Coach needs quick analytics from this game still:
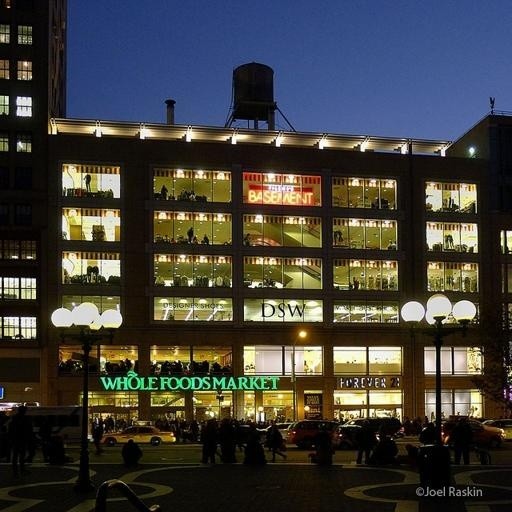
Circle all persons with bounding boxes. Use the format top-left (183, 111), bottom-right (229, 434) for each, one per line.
top-left (416, 424), bottom-right (469, 512)
top-left (85, 174), bottom-right (92, 193)
top-left (160, 185), bottom-right (169, 200)
top-left (188, 227), bottom-right (194, 244)
top-left (1, 402), bottom-right (472, 472)
top-left (106, 356), bottom-right (232, 379)
top-left (204, 234), bottom-right (210, 244)
top-left (243, 233), bottom-right (253, 247)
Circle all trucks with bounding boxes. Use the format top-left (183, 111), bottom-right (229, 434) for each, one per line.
top-left (0, 404), bottom-right (87, 449)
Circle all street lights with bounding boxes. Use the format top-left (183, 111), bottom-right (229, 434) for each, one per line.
top-left (292, 328), bottom-right (310, 421)
top-left (48, 301), bottom-right (125, 496)
top-left (401, 293), bottom-right (478, 444)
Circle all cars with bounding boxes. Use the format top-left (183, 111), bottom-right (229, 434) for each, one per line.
top-left (234, 416), bottom-right (409, 451)
top-left (417, 415), bottom-right (512, 447)
top-left (98, 420), bottom-right (177, 447)
